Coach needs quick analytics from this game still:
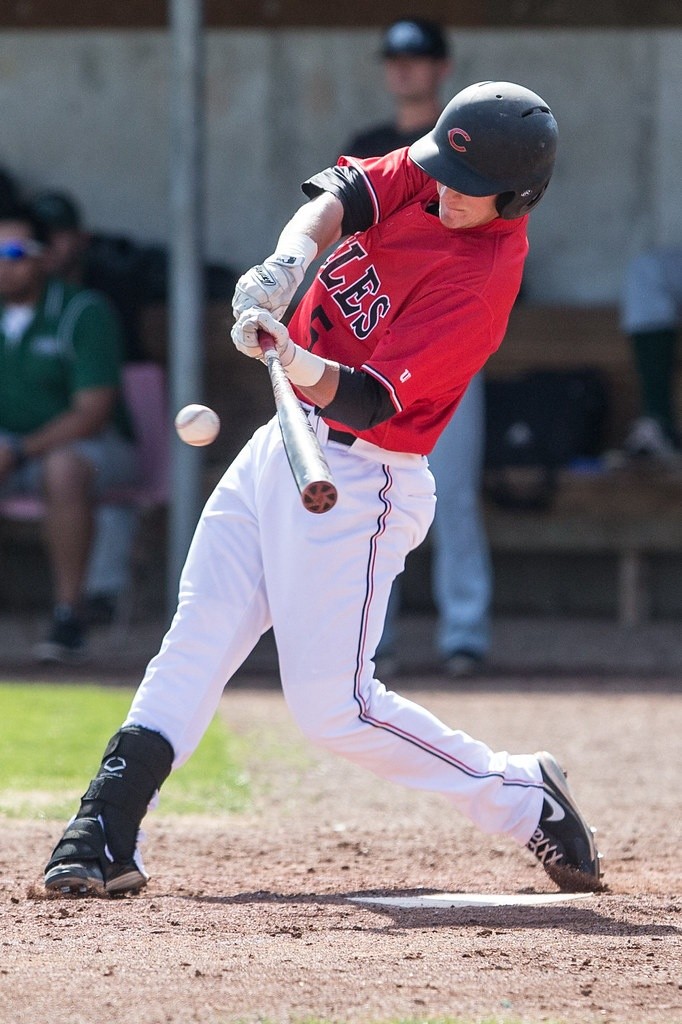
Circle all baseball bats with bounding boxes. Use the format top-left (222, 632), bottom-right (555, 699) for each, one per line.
top-left (256, 327), bottom-right (339, 516)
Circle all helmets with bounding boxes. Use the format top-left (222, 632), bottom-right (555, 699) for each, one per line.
top-left (406, 81), bottom-right (559, 220)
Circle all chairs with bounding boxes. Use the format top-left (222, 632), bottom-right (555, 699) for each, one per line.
top-left (0, 363), bottom-right (169, 639)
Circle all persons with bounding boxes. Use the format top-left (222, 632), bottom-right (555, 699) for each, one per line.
top-left (619, 233), bottom-right (682, 471)
top-left (0, 156), bottom-right (258, 674)
top-left (41, 78), bottom-right (604, 899)
top-left (333, 16), bottom-right (495, 678)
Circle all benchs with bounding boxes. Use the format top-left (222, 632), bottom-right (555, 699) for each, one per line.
top-left (138, 300), bottom-right (682, 627)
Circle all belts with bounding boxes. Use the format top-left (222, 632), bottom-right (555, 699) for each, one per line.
top-left (303, 409), bottom-right (356, 446)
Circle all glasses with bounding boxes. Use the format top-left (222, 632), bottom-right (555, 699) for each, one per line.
top-left (3, 241), bottom-right (43, 265)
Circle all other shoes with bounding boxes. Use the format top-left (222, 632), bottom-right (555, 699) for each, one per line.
top-left (31, 613), bottom-right (97, 667)
top-left (445, 649), bottom-right (483, 681)
top-left (374, 648), bottom-right (410, 681)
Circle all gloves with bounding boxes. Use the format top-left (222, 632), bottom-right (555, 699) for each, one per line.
top-left (230, 252), bottom-right (306, 318)
top-left (231, 307), bottom-right (298, 376)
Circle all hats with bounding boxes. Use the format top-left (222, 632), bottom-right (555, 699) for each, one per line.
top-left (376, 17), bottom-right (450, 62)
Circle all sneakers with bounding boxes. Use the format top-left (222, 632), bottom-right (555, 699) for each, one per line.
top-left (509, 750), bottom-right (604, 892)
top-left (44, 725), bottom-right (174, 894)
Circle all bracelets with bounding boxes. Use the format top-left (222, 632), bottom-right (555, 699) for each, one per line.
top-left (282, 343), bottom-right (325, 386)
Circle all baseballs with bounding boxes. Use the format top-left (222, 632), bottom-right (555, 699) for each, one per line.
top-left (173, 404), bottom-right (220, 448)
